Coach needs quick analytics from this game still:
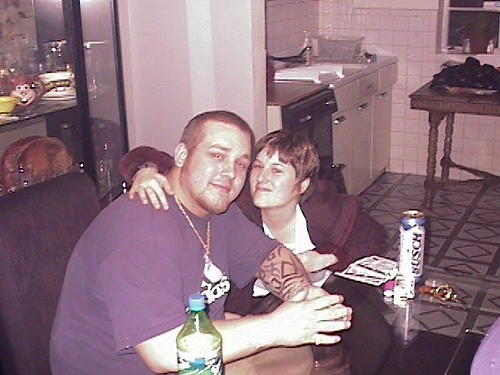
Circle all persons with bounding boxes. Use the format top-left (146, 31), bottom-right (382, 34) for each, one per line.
top-left (118, 130), bottom-right (394, 375)
top-left (49, 109), bottom-right (354, 375)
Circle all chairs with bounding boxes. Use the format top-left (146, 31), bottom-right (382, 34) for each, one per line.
top-left (1, 173), bottom-right (103, 375)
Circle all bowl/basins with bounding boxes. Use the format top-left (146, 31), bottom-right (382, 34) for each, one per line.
top-left (37, 72), bottom-right (70, 98)
top-left (0, 96), bottom-right (23, 113)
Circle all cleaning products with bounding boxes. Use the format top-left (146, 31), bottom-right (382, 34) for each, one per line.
top-left (301, 30), bottom-right (312, 66)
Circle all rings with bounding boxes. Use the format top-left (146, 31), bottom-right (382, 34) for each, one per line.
top-left (316, 333), bottom-right (321, 346)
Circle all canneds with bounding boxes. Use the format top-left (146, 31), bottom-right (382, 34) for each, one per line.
top-left (397, 209), bottom-right (426, 279)
top-left (395, 284), bottom-right (421, 345)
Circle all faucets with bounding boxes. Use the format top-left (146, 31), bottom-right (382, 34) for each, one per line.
top-left (286, 47), bottom-right (311, 67)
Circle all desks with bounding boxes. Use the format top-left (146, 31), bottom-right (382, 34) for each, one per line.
top-left (409, 82), bottom-right (500, 210)
top-left (171, 258), bottom-right (500, 374)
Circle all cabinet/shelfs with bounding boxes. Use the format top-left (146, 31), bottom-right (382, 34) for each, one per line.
top-left (0, 0), bottom-right (130, 208)
top-left (267, 63), bottom-right (398, 196)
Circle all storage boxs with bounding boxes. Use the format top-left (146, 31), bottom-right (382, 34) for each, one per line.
top-left (307, 34), bottom-right (364, 62)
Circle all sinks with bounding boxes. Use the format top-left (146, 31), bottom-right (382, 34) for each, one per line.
top-left (272, 61), bottom-right (367, 85)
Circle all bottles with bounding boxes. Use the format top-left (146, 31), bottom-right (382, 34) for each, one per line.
top-left (175, 293), bottom-right (224, 375)
top-left (463, 39), bottom-right (471, 54)
top-left (486, 40), bottom-right (494, 53)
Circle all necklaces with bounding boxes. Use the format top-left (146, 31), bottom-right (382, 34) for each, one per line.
top-left (173, 194), bottom-right (223, 283)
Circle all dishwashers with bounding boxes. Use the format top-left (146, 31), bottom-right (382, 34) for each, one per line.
top-left (281, 89), bottom-right (348, 198)
